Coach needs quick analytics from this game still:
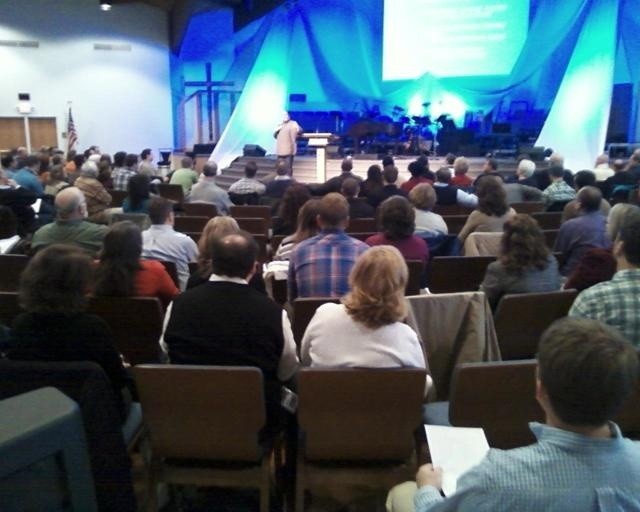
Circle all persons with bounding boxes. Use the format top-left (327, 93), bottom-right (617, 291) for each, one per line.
top-left (1, 111), bottom-right (640, 512)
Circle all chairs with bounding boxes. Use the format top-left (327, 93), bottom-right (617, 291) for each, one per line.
top-left (297, 367), bottom-right (419, 508)
top-left (425, 360), bottom-right (538, 449)
top-left (134, 367), bottom-right (271, 508)
top-left (0, 360), bottom-right (142, 511)
